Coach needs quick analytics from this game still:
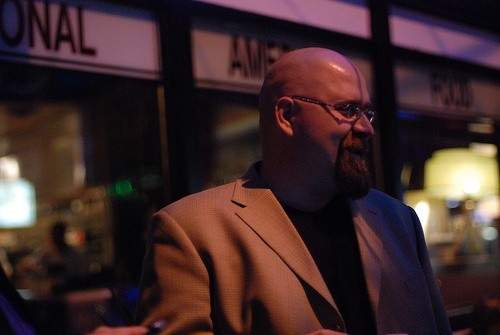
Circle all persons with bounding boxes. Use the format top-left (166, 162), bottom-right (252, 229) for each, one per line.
top-left (138, 46), bottom-right (457, 335)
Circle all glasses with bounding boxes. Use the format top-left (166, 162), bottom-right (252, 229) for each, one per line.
top-left (276, 95), bottom-right (378, 124)
